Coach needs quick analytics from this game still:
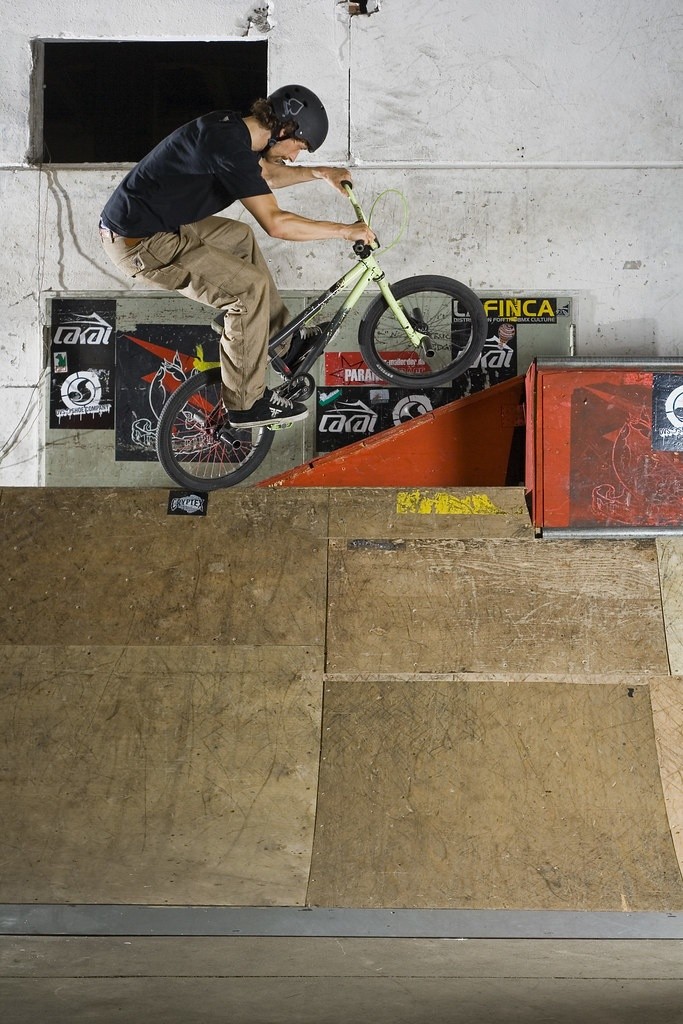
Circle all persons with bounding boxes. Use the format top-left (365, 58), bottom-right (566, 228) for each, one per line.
top-left (95, 86), bottom-right (379, 429)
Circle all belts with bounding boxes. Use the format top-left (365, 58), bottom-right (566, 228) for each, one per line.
top-left (99, 228), bottom-right (122, 238)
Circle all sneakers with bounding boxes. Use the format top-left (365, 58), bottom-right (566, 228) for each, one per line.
top-left (270, 321), bottom-right (340, 375)
top-left (228, 386), bottom-right (309, 428)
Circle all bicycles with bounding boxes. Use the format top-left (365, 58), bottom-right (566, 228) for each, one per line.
top-left (156, 166), bottom-right (489, 493)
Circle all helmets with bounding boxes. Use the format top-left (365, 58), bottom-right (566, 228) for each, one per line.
top-left (268, 85), bottom-right (329, 153)
